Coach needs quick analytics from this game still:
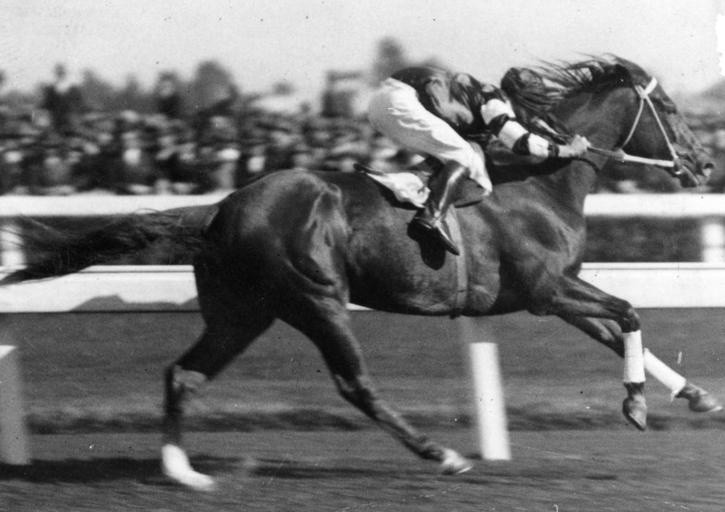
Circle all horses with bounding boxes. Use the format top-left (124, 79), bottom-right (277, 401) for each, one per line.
top-left (0, 50), bottom-right (725, 492)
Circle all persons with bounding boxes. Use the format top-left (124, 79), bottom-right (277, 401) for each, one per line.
top-left (587, 113), bottom-right (725, 193)
top-left (367, 66), bottom-right (592, 255)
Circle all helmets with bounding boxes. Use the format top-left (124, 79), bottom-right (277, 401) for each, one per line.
top-left (500, 65), bottom-right (546, 113)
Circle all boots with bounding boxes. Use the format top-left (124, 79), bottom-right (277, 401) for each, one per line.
top-left (408, 159), bottom-right (472, 257)
